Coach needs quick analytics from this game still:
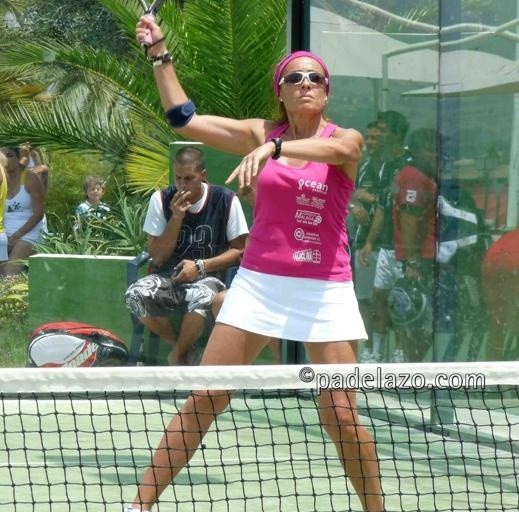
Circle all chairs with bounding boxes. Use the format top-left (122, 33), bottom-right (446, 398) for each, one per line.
top-left (126, 250), bottom-right (241, 366)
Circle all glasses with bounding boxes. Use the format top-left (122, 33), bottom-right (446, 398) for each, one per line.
top-left (278, 70), bottom-right (326, 87)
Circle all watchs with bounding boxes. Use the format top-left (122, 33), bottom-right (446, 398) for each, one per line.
top-left (271, 137), bottom-right (283, 159)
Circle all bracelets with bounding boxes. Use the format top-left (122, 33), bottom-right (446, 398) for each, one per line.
top-left (149, 49), bottom-right (172, 68)
top-left (194, 259), bottom-right (209, 278)
top-left (146, 37), bottom-right (165, 50)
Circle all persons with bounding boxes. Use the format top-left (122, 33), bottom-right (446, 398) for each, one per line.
top-left (0, 147), bottom-right (49, 282)
top-left (73, 175), bottom-right (112, 230)
top-left (213, 289), bottom-right (282, 363)
top-left (122, 10), bottom-right (390, 511)
top-left (18, 140), bottom-right (50, 175)
top-left (347, 107), bottom-right (518, 426)
top-left (0, 151), bottom-right (10, 270)
top-left (123, 145), bottom-right (252, 373)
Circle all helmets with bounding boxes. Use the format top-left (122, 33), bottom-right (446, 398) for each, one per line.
top-left (386, 278), bottom-right (430, 333)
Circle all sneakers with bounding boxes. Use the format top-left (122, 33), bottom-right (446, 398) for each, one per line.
top-left (359, 349), bottom-right (405, 363)
